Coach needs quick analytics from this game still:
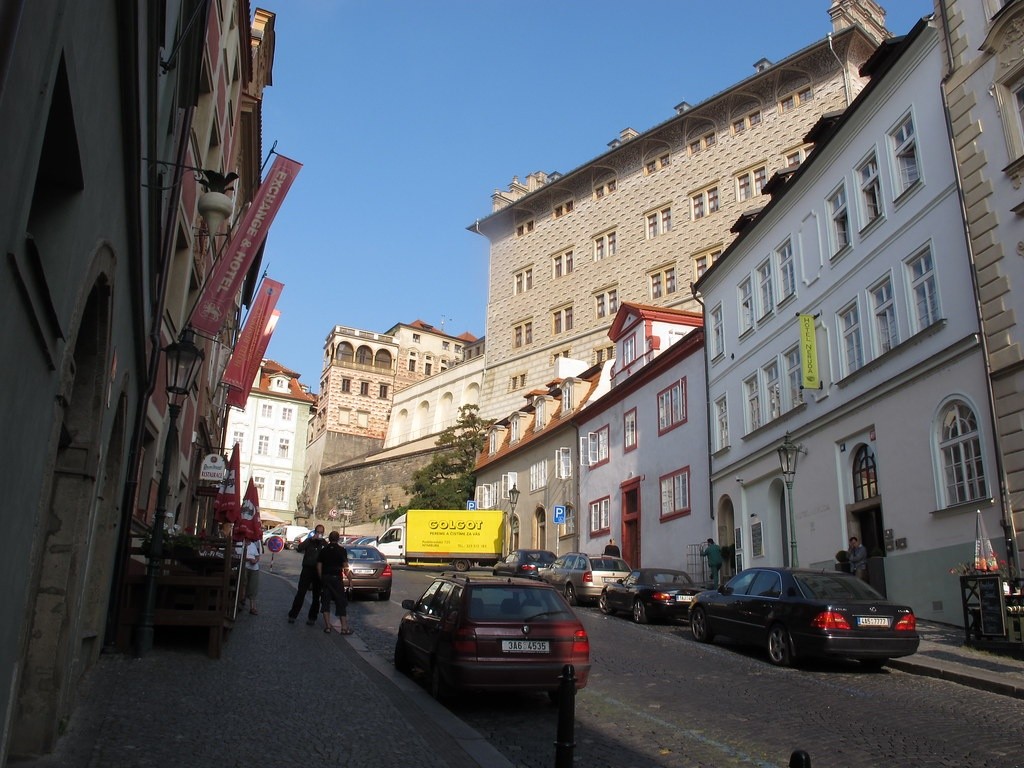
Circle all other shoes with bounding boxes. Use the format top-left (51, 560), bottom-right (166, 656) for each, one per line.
top-left (288, 616), bottom-right (296, 623)
top-left (250, 608), bottom-right (259, 615)
top-left (307, 620), bottom-right (316, 625)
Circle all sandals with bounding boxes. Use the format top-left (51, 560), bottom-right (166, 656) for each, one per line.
top-left (325, 627), bottom-right (331, 633)
top-left (341, 628), bottom-right (354, 635)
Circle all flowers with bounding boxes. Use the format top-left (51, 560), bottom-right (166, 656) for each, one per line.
top-left (947, 552), bottom-right (1015, 577)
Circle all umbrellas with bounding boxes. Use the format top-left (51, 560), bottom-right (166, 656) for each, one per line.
top-left (215, 442), bottom-right (263, 619)
top-left (973, 509), bottom-right (998, 574)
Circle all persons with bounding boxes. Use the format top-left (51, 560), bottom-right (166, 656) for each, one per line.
top-left (316, 531), bottom-right (355, 635)
top-left (604, 539), bottom-right (620, 559)
top-left (288, 525), bottom-right (328, 625)
top-left (699, 538), bottom-right (722, 591)
top-left (238, 541), bottom-right (260, 616)
top-left (846, 536), bottom-right (868, 581)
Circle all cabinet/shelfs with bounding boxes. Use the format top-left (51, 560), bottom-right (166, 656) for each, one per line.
top-left (119, 534), bottom-right (230, 660)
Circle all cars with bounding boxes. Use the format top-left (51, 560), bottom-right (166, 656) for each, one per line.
top-left (339, 543), bottom-right (392, 600)
top-left (537, 552), bottom-right (633, 607)
top-left (598, 568), bottom-right (707, 624)
top-left (492, 549), bottom-right (558, 582)
top-left (391, 572), bottom-right (591, 709)
top-left (689, 566), bottom-right (921, 676)
top-left (262, 524), bottom-right (375, 550)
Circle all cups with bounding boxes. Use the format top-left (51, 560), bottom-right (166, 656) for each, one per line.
top-left (316, 534), bottom-right (322, 540)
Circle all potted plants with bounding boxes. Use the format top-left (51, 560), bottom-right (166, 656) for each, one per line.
top-left (198, 169), bottom-right (238, 242)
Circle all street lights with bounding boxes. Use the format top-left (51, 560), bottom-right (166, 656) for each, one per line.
top-left (777, 431), bottom-right (800, 566)
top-left (382, 495), bottom-right (391, 531)
top-left (129, 320), bottom-right (206, 660)
top-left (507, 483), bottom-right (521, 551)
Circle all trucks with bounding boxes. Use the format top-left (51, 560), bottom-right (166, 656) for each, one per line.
top-left (367, 509), bottom-right (507, 572)
